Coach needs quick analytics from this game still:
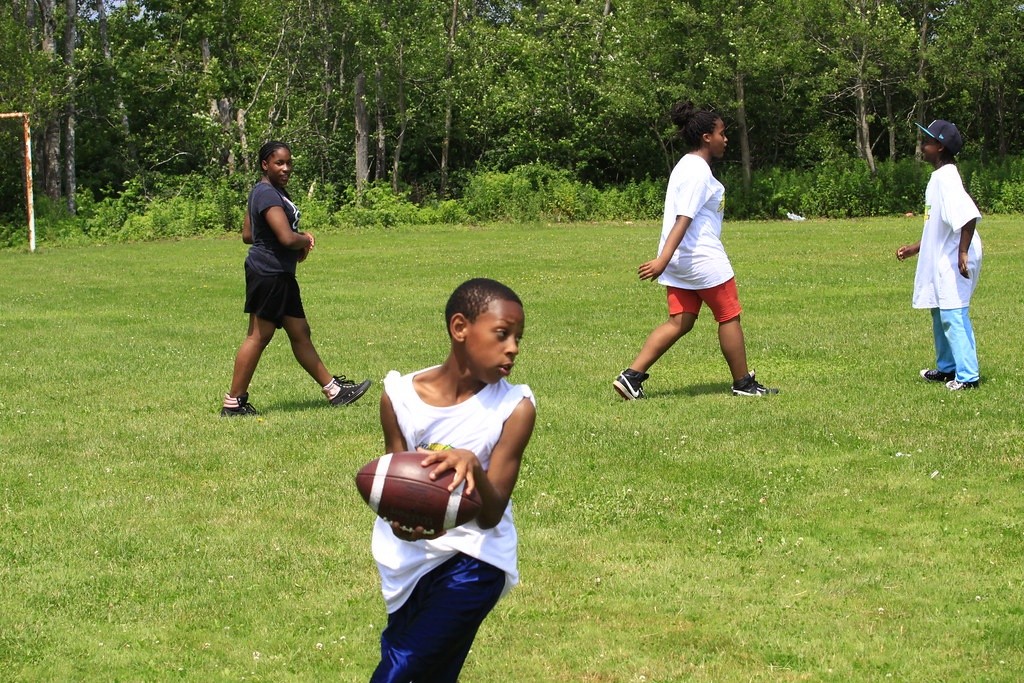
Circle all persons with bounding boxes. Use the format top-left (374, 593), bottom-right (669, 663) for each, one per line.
top-left (896, 121), bottom-right (983, 392)
top-left (611, 100), bottom-right (778, 400)
top-left (367, 278), bottom-right (537, 682)
top-left (219, 141), bottom-right (370, 416)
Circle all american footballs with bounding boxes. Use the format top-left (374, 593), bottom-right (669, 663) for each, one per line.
top-left (354, 452), bottom-right (482, 535)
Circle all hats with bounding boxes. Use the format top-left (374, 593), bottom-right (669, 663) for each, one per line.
top-left (915, 119), bottom-right (966, 156)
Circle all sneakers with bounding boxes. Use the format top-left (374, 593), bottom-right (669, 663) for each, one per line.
top-left (612, 369), bottom-right (648, 401)
top-left (323, 376), bottom-right (373, 407)
top-left (920, 367), bottom-right (956, 382)
top-left (943, 380), bottom-right (980, 389)
top-left (219, 393), bottom-right (260, 417)
top-left (734, 369), bottom-right (779, 397)
